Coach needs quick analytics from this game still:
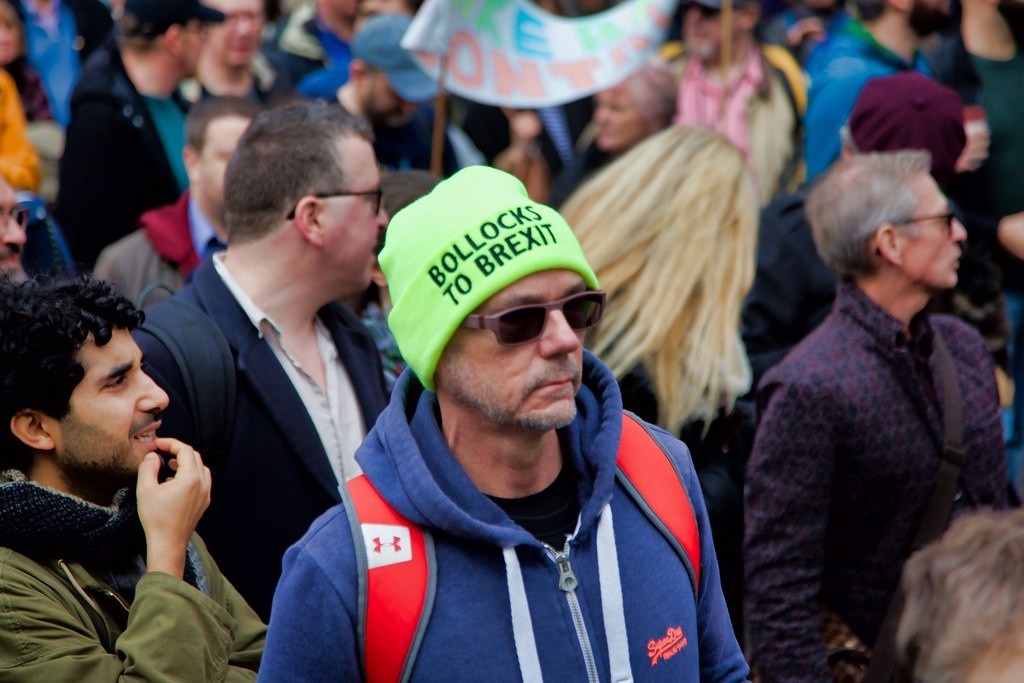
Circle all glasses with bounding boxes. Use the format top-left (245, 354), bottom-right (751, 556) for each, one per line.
top-left (683, 0), bottom-right (720, 16)
top-left (287, 185), bottom-right (384, 220)
top-left (11, 208), bottom-right (30, 231)
top-left (874, 198), bottom-right (959, 256)
top-left (461, 287), bottom-right (607, 347)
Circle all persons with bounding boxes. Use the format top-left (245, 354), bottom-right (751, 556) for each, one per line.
top-left (557, 124), bottom-right (754, 652)
top-left (0, 275), bottom-right (269, 683)
top-left (254, 164), bottom-right (750, 683)
top-left (131, 101), bottom-right (391, 626)
top-left (0, 0), bottom-right (1024, 410)
top-left (743, 150), bottom-right (1022, 683)
top-left (894, 504), bottom-right (1024, 683)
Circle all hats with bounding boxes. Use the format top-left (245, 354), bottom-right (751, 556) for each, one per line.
top-left (350, 13), bottom-right (448, 103)
top-left (123, 0), bottom-right (225, 39)
top-left (849, 71), bottom-right (967, 185)
top-left (390, 169), bottom-right (601, 394)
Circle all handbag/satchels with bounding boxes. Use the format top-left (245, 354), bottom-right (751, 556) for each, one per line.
top-left (740, 599), bottom-right (873, 683)
